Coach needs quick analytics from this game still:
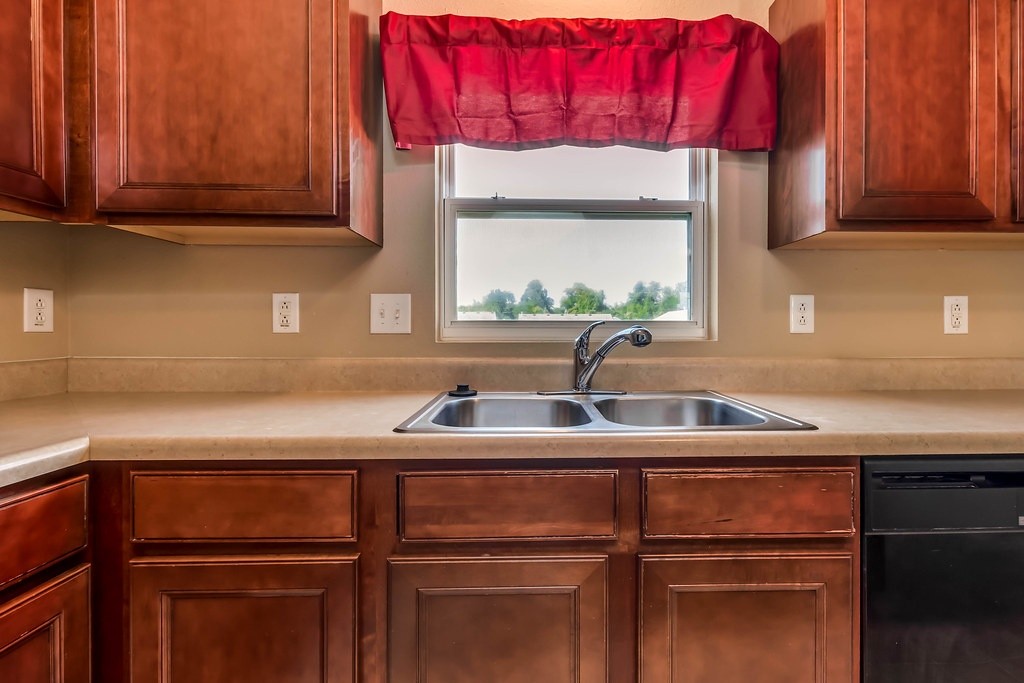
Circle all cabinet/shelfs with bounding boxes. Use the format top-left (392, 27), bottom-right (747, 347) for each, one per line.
top-left (767, 0), bottom-right (1024, 252)
top-left (118, 467), bottom-right (856, 683)
top-left (0, 0), bottom-right (384, 248)
top-left (0, 473), bottom-right (93, 683)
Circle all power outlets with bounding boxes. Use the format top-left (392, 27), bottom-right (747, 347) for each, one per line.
top-left (943, 296), bottom-right (968, 335)
top-left (272, 292), bottom-right (299, 334)
top-left (789, 295), bottom-right (814, 334)
top-left (371, 293), bottom-right (412, 334)
top-left (23, 287), bottom-right (54, 333)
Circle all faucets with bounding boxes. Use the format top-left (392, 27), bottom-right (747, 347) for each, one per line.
top-left (573, 320), bottom-right (652, 391)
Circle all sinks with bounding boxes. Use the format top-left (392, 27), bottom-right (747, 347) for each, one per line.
top-left (432, 396), bottom-right (591, 426)
top-left (595, 396), bottom-right (768, 428)
